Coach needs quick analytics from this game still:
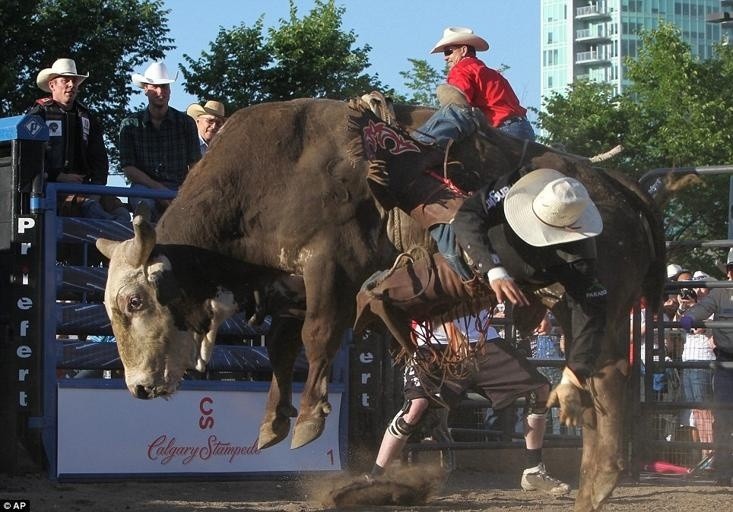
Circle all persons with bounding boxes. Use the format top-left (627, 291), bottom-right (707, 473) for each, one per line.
top-left (451, 149), bottom-right (666, 423)
top-left (530, 258), bottom-right (733, 488)
top-left (20, 59), bottom-right (133, 223)
top-left (115, 62), bottom-right (199, 222)
top-left (185, 101), bottom-right (227, 156)
top-left (358, 302), bottom-right (570, 495)
top-left (431, 28), bottom-right (532, 170)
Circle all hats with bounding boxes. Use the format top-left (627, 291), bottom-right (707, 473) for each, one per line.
top-left (186, 100), bottom-right (229, 124)
top-left (36, 58), bottom-right (89, 94)
top-left (502, 168), bottom-right (604, 248)
top-left (429, 26), bottom-right (490, 55)
top-left (130, 61), bottom-right (179, 89)
top-left (667, 263), bottom-right (717, 282)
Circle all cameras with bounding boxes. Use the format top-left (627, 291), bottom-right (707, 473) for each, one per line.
top-left (678, 290), bottom-right (698, 304)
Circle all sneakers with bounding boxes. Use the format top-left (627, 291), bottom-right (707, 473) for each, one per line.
top-left (434, 83), bottom-right (480, 129)
top-left (519, 463), bottom-right (572, 496)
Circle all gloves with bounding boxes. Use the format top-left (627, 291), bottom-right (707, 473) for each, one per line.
top-left (544, 366), bottom-right (590, 429)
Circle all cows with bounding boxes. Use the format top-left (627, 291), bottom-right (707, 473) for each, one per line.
top-left (95, 96), bottom-right (665, 512)
